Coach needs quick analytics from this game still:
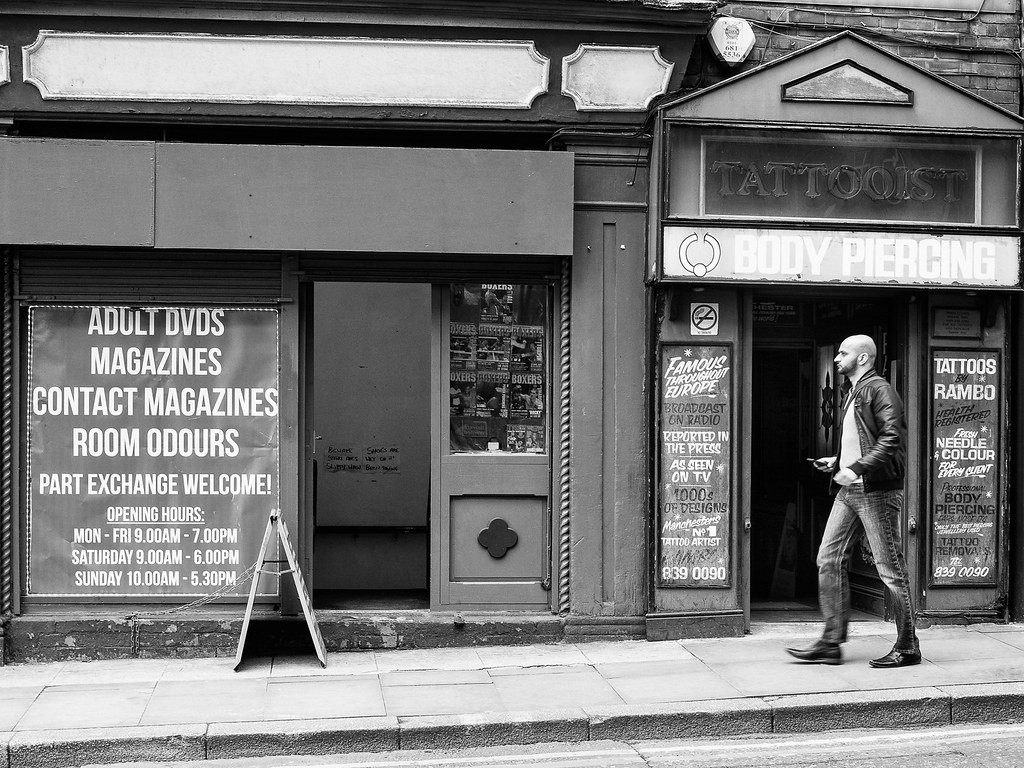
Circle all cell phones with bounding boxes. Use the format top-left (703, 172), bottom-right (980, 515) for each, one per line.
top-left (806, 458), bottom-right (834, 468)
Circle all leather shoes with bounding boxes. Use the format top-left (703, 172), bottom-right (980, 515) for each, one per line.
top-left (784, 641), bottom-right (843, 663)
top-left (868, 649), bottom-right (922, 668)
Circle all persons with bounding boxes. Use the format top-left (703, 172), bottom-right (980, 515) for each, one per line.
top-left (785, 335), bottom-right (922, 668)
